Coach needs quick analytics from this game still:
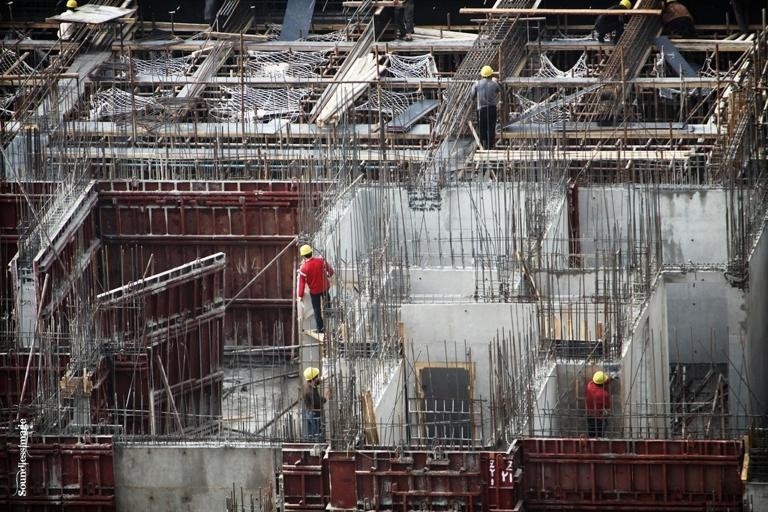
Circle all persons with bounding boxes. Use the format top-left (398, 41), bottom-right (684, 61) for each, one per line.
top-left (663, 0), bottom-right (698, 37)
top-left (471, 66), bottom-right (501, 150)
top-left (393, 0), bottom-right (415, 41)
top-left (57, 0), bottom-right (78, 42)
top-left (298, 245), bottom-right (333, 333)
top-left (587, 371), bottom-right (612, 437)
top-left (595, 0), bottom-right (632, 44)
top-left (303, 367), bottom-right (326, 443)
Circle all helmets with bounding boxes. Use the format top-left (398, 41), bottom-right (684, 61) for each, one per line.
top-left (620, 0), bottom-right (632, 9)
top-left (304, 367), bottom-right (320, 381)
top-left (480, 65), bottom-right (493, 77)
top-left (300, 245), bottom-right (313, 257)
top-left (67, 0), bottom-right (77, 8)
top-left (593, 370), bottom-right (607, 384)
